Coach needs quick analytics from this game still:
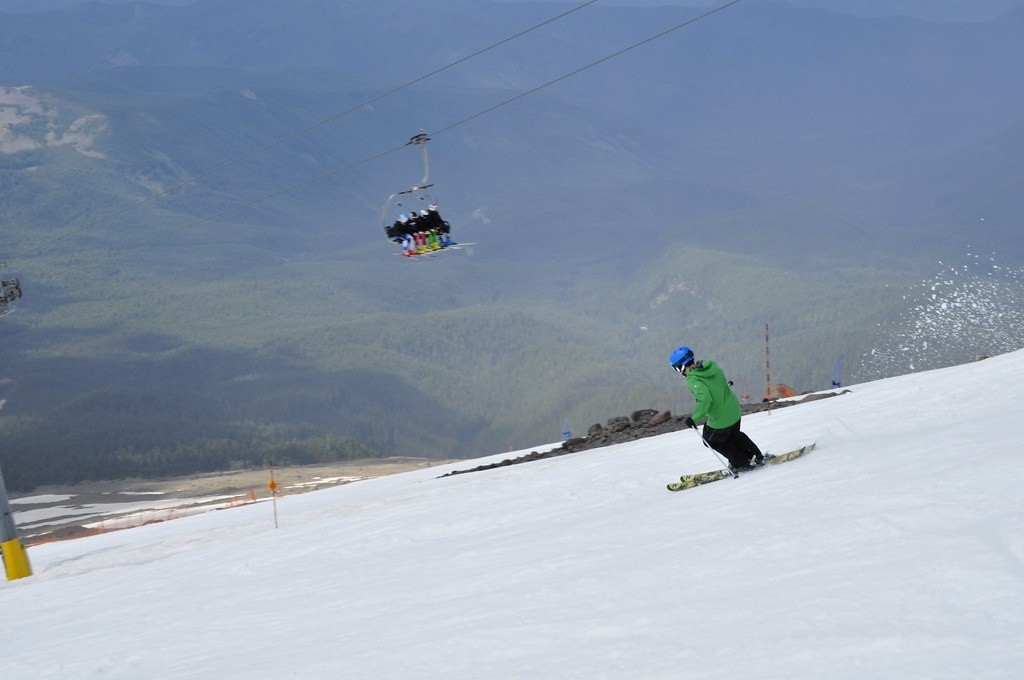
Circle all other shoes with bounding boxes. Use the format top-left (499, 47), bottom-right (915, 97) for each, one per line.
top-left (753, 454), bottom-right (764, 465)
top-left (403, 238), bottom-right (457, 256)
top-left (724, 461), bottom-right (749, 475)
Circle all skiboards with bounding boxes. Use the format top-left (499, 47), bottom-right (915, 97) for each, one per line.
top-left (393, 242), bottom-right (478, 261)
top-left (667, 442), bottom-right (816, 492)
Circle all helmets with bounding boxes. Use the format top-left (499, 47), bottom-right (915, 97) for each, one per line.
top-left (400, 203), bottom-right (438, 222)
top-left (668, 347), bottom-right (694, 368)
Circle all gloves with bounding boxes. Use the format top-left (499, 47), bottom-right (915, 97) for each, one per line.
top-left (685, 417), bottom-right (695, 427)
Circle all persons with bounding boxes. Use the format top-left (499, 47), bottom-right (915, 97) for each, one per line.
top-left (668, 346), bottom-right (764, 472)
top-left (417, 209), bottom-right (443, 250)
top-left (392, 214), bottom-right (417, 257)
top-left (407, 211), bottom-right (432, 254)
top-left (428, 203), bottom-right (457, 247)
